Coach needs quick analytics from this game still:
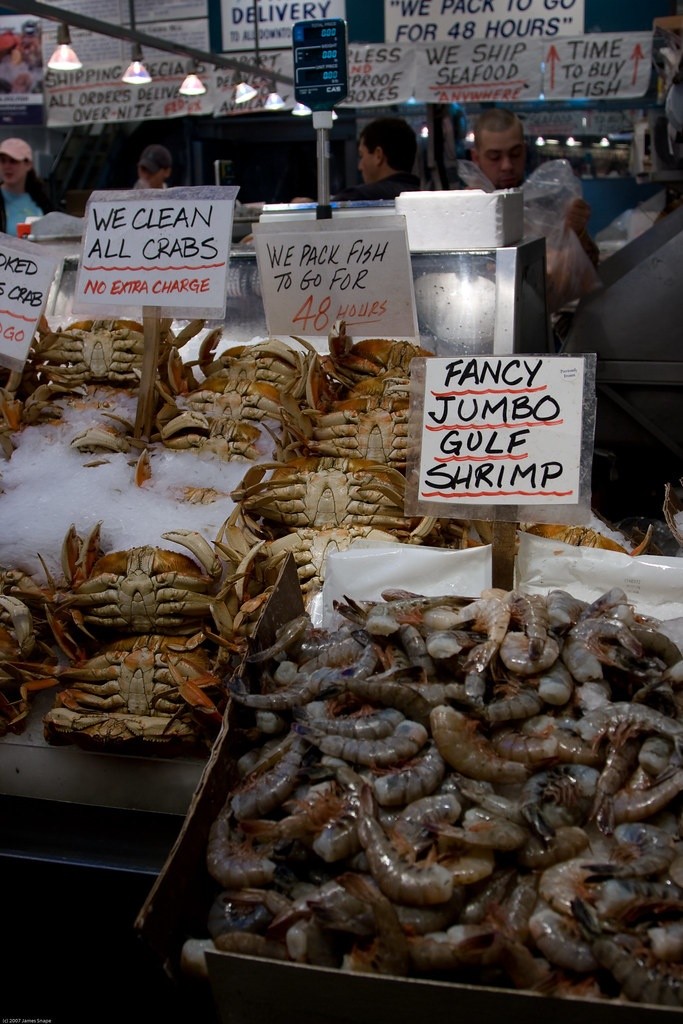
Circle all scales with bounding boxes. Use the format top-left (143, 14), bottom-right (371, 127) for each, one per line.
top-left (250, 19), bottom-right (396, 221)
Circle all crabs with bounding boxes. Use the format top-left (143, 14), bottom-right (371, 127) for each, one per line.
top-left (0, 315), bottom-right (206, 467)
top-left (520, 523), bottom-right (657, 556)
top-left (1, 520), bottom-right (233, 757)
top-left (208, 571), bottom-right (278, 653)
top-left (165, 323), bottom-right (494, 599)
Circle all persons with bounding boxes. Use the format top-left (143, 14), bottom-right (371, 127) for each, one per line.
top-left (131, 144), bottom-right (172, 190)
top-left (289, 115), bottom-right (422, 204)
top-left (0, 137), bottom-right (57, 241)
top-left (653, 52), bottom-right (683, 226)
top-left (456, 106), bottom-right (606, 355)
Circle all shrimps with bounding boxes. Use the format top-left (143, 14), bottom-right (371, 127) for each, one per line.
top-left (206, 589), bottom-right (683, 1009)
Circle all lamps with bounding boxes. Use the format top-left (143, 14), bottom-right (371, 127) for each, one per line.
top-left (179, 64), bottom-right (207, 95)
top-left (121, 46), bottom-right (153, 85)
top-left (48, 27), bottom-right (83, 71)
top-left (263, 87), bottom-right (285, 110)
top-left (291, 102), bottom-right (313, 116)
top-left (234, 76), bottom-right (257, 104)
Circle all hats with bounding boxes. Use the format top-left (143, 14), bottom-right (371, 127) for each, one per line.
top-left (138, 145), bottom-right (171, 173)
top-left (0, 137), bottom-right (32, 162)
